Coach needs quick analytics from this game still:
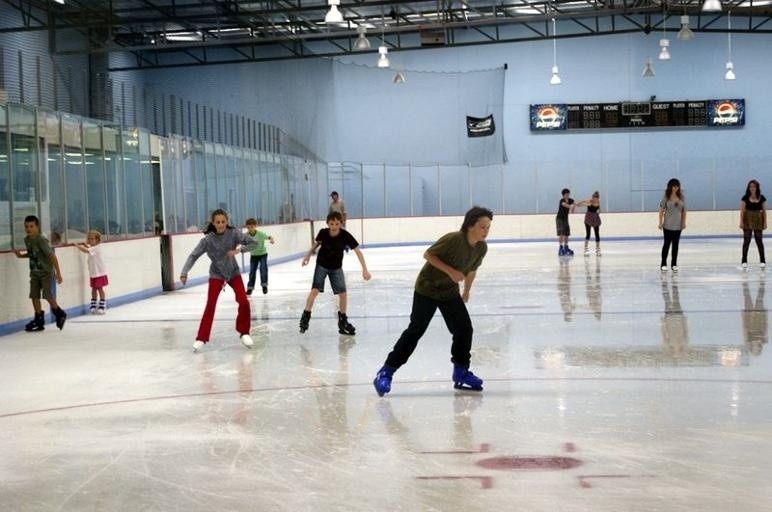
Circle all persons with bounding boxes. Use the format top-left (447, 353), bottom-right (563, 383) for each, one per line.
top-left (298, 210), bottom-right (374, 336)
top-left (554, 187), bottom-right (579, 257)
top-left (370, 203), bottom-right (495, 400)
top-left (280, 192), bottom-right (297, 223)
top-left (242, 216), bottom-right (276, 297)
top-left (656, 176), bottom-right (688, 272)
top-left (575, 190), bottom-right (604, 257)
top-left (326, 189), bottom-right (350, 254)
top-left (178, 207), bottom-right (260, 353)
top-left (72, 228), bottom-right (111, 315)
top-left (737, 178), bottom-right (769, 270)
top-left (69, 198), bottom-right (89, 227)
top-left (12, 214), bottom-right (69, 335)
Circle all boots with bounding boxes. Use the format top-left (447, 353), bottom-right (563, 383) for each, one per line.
top-left (25, 311), bottom-right (45, 331)
top-left (51, 305), bottom-right (66, 329)
top-left (337, 311), bottom-right (355, 334)
top-left (564, 245), bottom-right (573, 255)
top-left (300, 310), bottom-right (311, 330)
top-left (559, 245), bottom-right (565, 256)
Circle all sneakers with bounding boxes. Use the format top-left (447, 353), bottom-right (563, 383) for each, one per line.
top-left (98, 309), bottom-right (105, 314)
top-left (662, 266), bottom-right (668, 271)
top-left (90, 307), bottom-right (96, 313)
top-left (761, 263), bottom-right (765, 268)
top-left (193, 340), bottom-right (204, 350)
top-left (241, 334), bottom-right (252, 346)
top-left (376, 366), bottom-right (394, 394)
top-left (673, 266), bottom-right (679, 272)
top-left (263, 285), bottom-right (267, 294)
top-left (246, 289), bottom-right (252, 295)
top-left (743, 263), bottom-right (747, 268)
top-left (452, 367), bottom-right (483, 387)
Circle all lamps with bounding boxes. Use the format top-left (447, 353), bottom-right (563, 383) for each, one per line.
top-left (392, 68), bottom-right (408, 85)
top-left (724, 10), bottom-right (737, 80)
top-left (657, 0), bottom-right (672, 60)
top-left (377, 1), bottom-right (390, 69)
top-left (640, 1), bottom-right (655, 78)
top-left (353, 24), bottom-right (372, 49)
top-left (676, 1), bottom-right (695, 42)
top-left (549, 19), bottom-right (562, 85)
top-left (700, 1), bottom-right (723, 12)
top-left (324, 0), bottom-right (344, 22)
